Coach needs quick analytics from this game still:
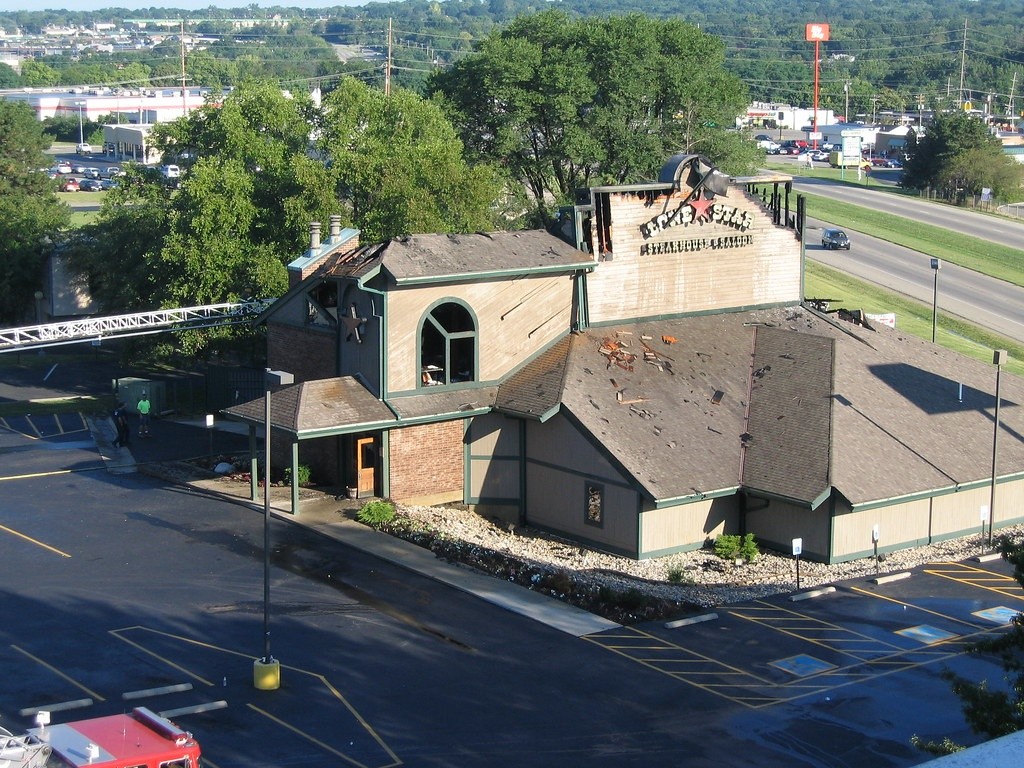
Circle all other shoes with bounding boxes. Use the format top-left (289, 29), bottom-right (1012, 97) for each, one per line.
top-left (120, 444), bottom-right (126, 447)
top-left (112, 441), bottom-right (117, 448)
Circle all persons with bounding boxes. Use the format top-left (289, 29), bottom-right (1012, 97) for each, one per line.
top-left (112, 402), bottom-right (129, 449)
top-left (137, 393), bottom-right (150, 434)
top-left (806, 153), bottom-right (812, 167)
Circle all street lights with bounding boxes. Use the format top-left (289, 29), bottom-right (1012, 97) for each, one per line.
top-left (931, 259), bottom-right (941, 343)
top-left (74, 101), bottom-right (86, 151)
top-left (261, 368), bottom-right (294, 663)
top-left (988, 349), bottom-right (1008, 544)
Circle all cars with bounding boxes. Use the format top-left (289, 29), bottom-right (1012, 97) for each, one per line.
top-left (167, 180), bottom-right (181, 189)
top-left (28, 160), bottom-right (145, 192)
top-left (754, 134), bottom-right (904, 168)
top-left (102, 141), bottom-right (115, 153)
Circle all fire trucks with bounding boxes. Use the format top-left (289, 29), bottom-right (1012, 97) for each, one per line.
top-left (0, 705), bottom-right (203, 768)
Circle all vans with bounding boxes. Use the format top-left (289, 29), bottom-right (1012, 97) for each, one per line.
top-left (160, 165), bottom-right (180, 180)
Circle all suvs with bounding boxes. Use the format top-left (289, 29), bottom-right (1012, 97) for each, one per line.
top-left (822, 228), bottom-right (850, 250)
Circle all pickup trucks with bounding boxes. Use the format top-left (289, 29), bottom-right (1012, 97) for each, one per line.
top-left (76, 143), bottom-right (92, 154)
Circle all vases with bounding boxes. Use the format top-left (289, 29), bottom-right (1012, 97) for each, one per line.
top-left (346, 485), bottom-right (358, 499)
